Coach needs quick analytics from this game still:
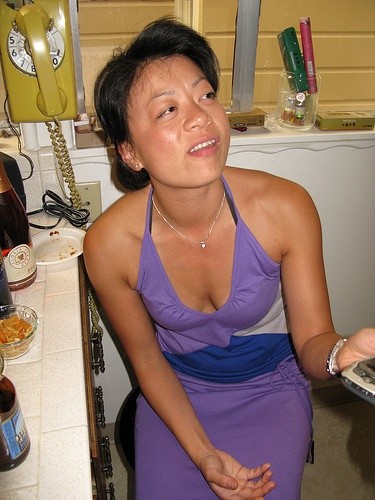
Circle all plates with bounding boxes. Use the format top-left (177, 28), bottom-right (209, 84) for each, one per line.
top-left (27, 228), bottom-right (87, 265)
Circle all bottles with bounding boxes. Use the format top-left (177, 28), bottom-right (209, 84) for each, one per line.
top-left (0, 151), bottom-right (37, 316)
top-left (0, 373), bottom-right (29, 472)
top-left (0, 248), bottom-right (15, 319)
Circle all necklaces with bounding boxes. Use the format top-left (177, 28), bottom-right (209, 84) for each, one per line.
top-left (151, 192), bottom-right (226, 248)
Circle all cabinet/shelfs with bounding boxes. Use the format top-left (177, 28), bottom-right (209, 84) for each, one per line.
top-left (0, 149), bottom-right (115, 500)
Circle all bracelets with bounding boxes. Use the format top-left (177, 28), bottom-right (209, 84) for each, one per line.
top-left (326, 338), bottom-right (348, 377)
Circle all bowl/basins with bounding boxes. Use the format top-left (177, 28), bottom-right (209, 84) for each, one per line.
top-left (0, 305), bottom-right (38, 359)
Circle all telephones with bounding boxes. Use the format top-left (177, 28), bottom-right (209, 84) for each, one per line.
top-left (0, 0), bottom-right (77, 124)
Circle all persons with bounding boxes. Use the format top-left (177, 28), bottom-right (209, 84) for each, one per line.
top-left (84, 16), bottom-right (375, 500)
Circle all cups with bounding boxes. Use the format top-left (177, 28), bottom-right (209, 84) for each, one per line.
top-left (276, 73), bottom-right (320, 132)
